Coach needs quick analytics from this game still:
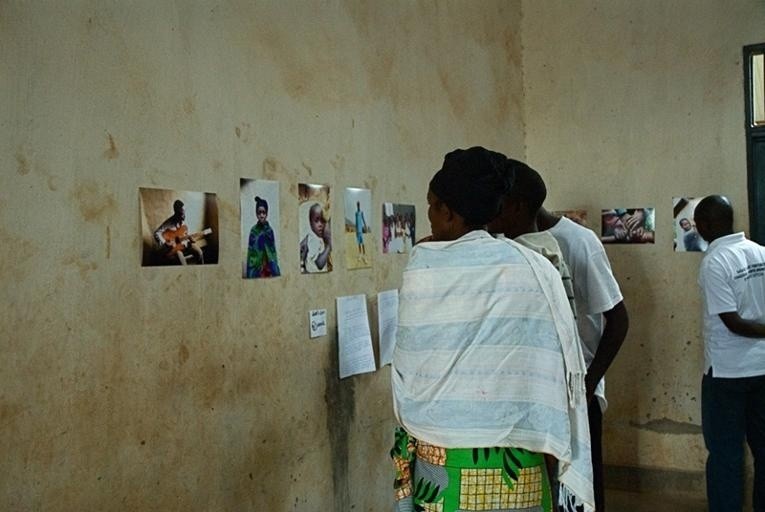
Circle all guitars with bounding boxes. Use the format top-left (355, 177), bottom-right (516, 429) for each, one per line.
top-left (162, 224), bottom-right (213, 257)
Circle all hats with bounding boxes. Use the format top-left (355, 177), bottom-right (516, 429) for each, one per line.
top-left (256, 200), bottom-right (268, 211)
top-left (429, 146), bottom-right (515, 225)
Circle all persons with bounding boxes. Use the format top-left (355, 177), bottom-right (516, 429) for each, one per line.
top-left (693, 194), bottom-right (765, 512)
top-left (536, 193), bottom-right (631, 512)
top-left (387, 147), bottom-right (583, 511)
top-left (300, 203), bottom-right (330, 271)
top-left (354, 201), bottom-right (369, 265)
top-left (602, 208), bottom-right (655, 243)
top-left (248, 196), bottom-right (278, 277)
top-left (489, 152), bottom-right (581, 321)
top-left (154, 199), bottom-right (203, 266)
top-left (383, 211), bottom-right (439, 253)
top-left (679, 217), bottom-right (704, 251)
